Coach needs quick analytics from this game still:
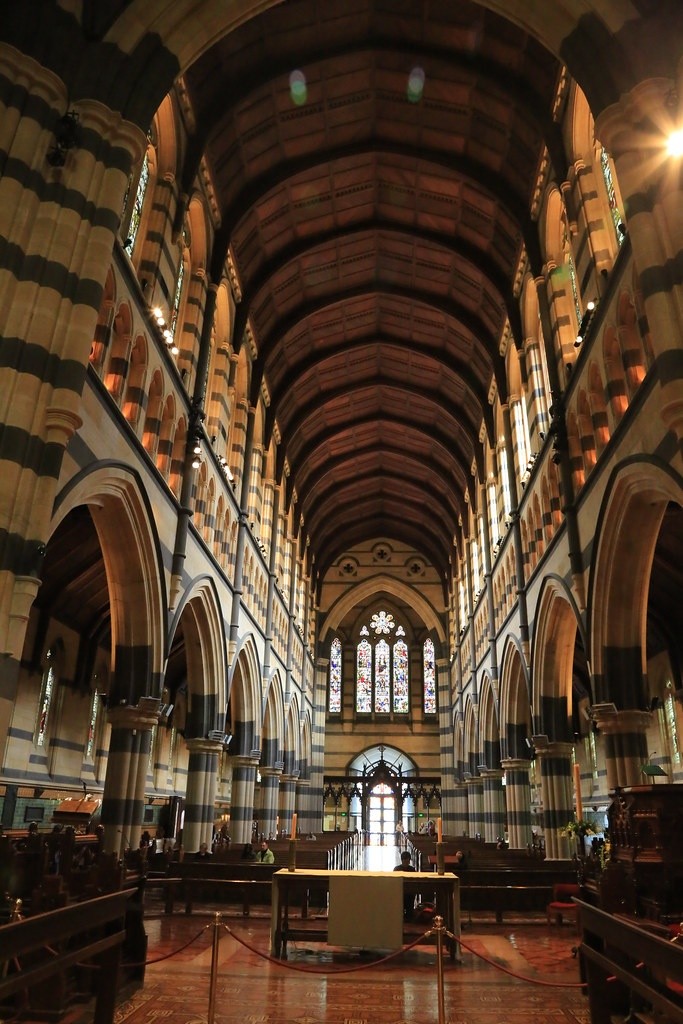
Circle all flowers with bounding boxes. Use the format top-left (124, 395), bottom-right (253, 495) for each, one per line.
top-left (559, 818), bottom-right (607, 840)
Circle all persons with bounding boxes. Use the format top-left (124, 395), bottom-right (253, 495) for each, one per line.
top-left (395, 821), bottom-right (404, 846)
top-left (355, 826), bottom-right (358, 833)
top-left (242, 841), bottom-right (274, 864)
top-left (306, 832), bottom-right (316, 840)
top-left (194, 843), bottom-right (212, 860)
top-left (429, 821), bottom-right (434, 836)
top-left (395, 851), bottom-right (419, 913)
top-left (456, 849), bottom-right (467, 870)
top-left (419, 823), bottom-right (426, 834)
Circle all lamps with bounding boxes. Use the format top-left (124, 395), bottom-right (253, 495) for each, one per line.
top-left (477, 765), bottom-right (488, 771)
top-left (532, 734), bottom-right (549, 747)
top-left (592, 703), bottom-right (619, 719)
top-left (249, 749), bottom-right (261, 760)
top-left (275, 761), bottom-right (284, 771)
top-left (208, 730), bottom-right (232, 745)
top-left (136, 695), bottom-right (174, 718)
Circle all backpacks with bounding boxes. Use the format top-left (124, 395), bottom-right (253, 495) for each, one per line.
top-left (413, 902), bottom-right (436, 922)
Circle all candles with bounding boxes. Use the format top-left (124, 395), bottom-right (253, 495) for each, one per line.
top-left (438, 817), bottom-right (442, 842)
top-left (291, 814), bottom-right (297, 839)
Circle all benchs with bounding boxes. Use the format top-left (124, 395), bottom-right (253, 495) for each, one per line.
top-left (401, 832), bottom-right (580, 924)
top-left (165, 832), bottom-right (363, 908)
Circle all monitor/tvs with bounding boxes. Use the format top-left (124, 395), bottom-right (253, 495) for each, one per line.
top-left (23, 807), bottom-right (45, 822)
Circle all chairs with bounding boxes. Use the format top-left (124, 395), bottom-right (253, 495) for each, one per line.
top-left (546, 883), bottom-right (580, 936)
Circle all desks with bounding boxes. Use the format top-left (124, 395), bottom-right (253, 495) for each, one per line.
top-left (271, 867), bottom-right (462, 965)
